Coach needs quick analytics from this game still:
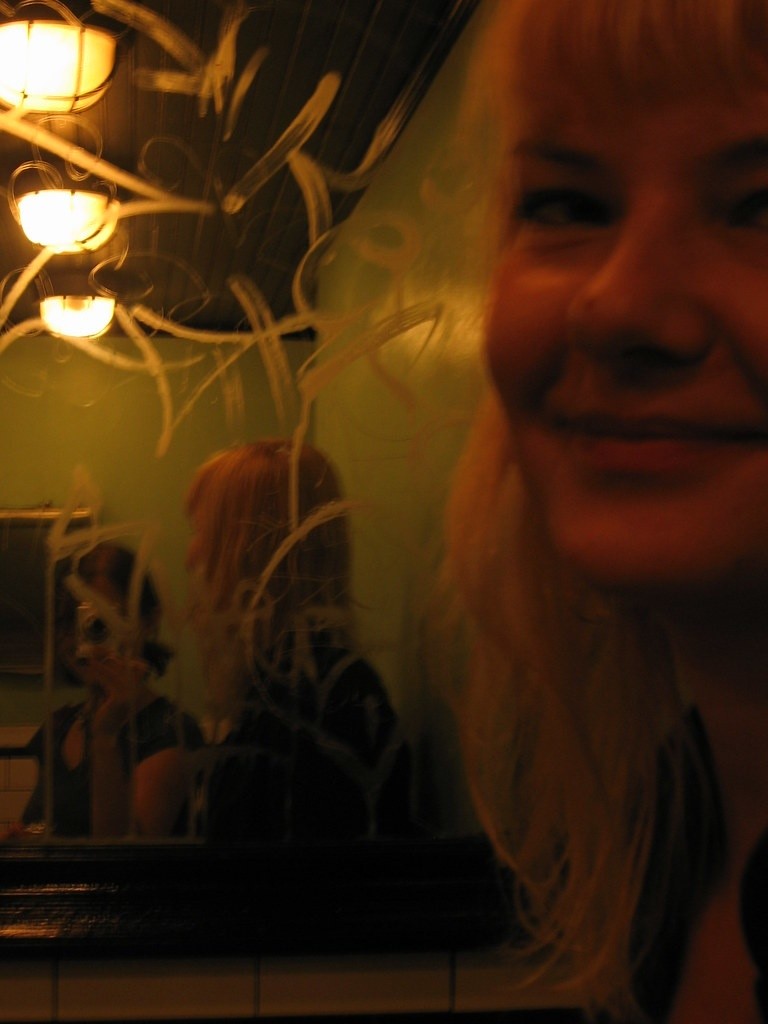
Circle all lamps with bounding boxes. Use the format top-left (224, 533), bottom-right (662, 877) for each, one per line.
top-left (0, 151), bottom-right (139, 255)
top-left (0, 0), bottom-right (123, 113)
top-left (31, 266), bottom-right (136, 342)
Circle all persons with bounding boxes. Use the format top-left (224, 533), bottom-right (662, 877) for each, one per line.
top-left (180, 438), bottom-right (409, 959)
top-left (23, 540), bottom-right (204, 838)
top-left (421, 0), bottom-right (768, 1024)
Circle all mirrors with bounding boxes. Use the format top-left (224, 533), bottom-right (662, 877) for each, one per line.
top-left (0, 506), bottom-right (100, 674)
top-left (0, 0), bottom-right (768, 1024)
